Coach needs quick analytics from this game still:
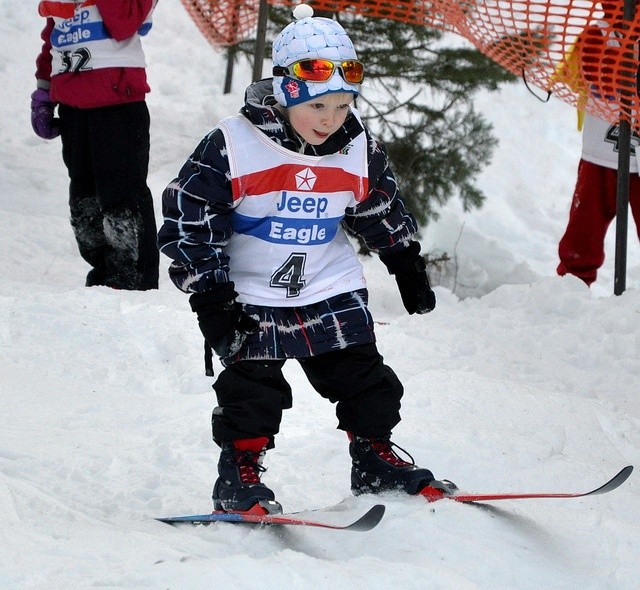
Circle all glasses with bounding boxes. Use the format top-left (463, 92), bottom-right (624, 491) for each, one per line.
top-left (272, 58), bottom-right (365, 85)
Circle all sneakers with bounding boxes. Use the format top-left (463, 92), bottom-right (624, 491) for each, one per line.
top-left (212, 436), bottom-right (275, 512)
top-left (347, 430), bottom-right (434, 496)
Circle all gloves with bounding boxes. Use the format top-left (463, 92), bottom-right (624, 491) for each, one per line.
top-left (379, 240), bottom-right (436, 315)
top-left (189, 281), bottom-right (260, 376)
top-left (31, 89), bottom-right (60, 139)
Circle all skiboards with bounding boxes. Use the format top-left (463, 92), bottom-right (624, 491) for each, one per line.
top-left (155, 466), bottom-right (634, 531)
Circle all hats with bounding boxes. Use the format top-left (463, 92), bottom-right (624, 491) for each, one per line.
top-left (271, 4), bottom-right (360, 108)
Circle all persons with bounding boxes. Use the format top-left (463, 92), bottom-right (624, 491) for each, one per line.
top-left (156, 0), bottom-right (435, 513)
top-left (31, 0), bottom-right (159, 292)
top-left (556, 1), bottom-right (639, 285)
